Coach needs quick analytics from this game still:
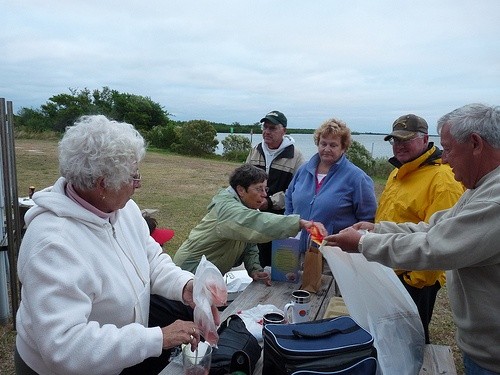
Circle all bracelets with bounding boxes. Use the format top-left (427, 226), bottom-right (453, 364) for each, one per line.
top-left (358, 235), bottom-right (365, 253)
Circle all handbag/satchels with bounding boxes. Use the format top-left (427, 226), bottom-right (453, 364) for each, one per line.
top-left (199, 314), bottom-right (262, 375)
top-left (261, 315), bottom-right (378, 375)
top-left (302, 233), bottom-right (323, 293)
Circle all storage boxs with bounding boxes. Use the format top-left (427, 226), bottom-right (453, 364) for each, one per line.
top-left (271, 228), bottom-right (306, 284)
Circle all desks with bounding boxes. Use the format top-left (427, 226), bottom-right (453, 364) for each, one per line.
top-left (156, 266), bottom-right (336, 375)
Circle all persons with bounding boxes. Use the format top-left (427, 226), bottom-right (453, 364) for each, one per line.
top-left (15, 115), bottom-right (220, 375)
top-left (141, 211), bottom-right (197, 375)
top-left (245, 110), bottom-right (305, 269)
top-left (373, 115), bottom-right (467, 344)
top-left (323, 104), bottom-right (500, 375)
top-left (173, 164), bottom-right (314, 287)
top-left (284, 117), bottom-right (377, 275)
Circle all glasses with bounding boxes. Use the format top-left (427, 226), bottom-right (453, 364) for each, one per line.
top-left (389, 137), bottom-right (420, 146)
top-left (131, 169), bottom-right (140, 183)
top-left (249, 187), bottom-right (269, 193)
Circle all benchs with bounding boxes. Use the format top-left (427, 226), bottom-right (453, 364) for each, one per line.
top-left (419, 344), bottom-right (457, 375)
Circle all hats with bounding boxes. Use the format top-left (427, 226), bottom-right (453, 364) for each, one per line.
top-left (384, 114), bottom-right (428, 141)
top-left (259, 110), bottom-right (287, 127)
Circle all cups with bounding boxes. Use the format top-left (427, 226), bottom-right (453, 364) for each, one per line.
top-left (182, 343), bottom-right (213, 375)
top-left (284, 290), bottom-right (312, 324)
top-left (262, 312), bottom-right (285, 342)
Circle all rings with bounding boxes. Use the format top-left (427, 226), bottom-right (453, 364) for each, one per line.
top-left (192, 327), bottom-right (195, 335)
top-left (190, 335), bottom-right (194, 342)
